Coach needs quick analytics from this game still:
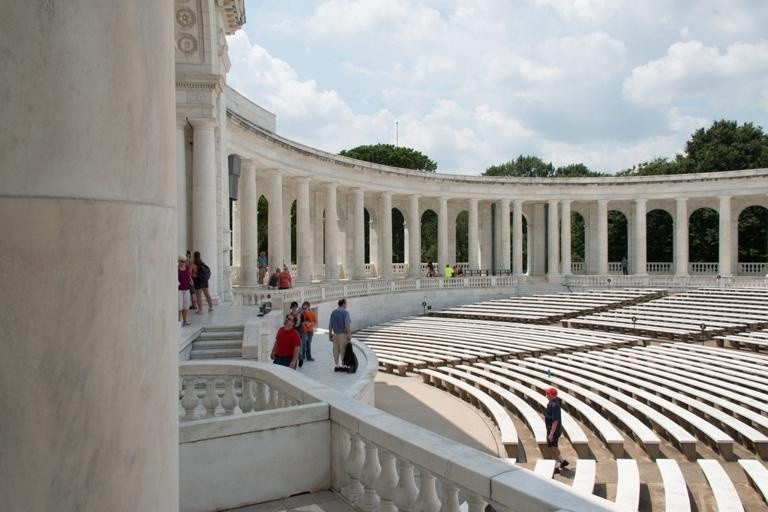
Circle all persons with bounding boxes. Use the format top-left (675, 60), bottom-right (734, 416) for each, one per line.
top-left (621, 256), bottom-right (629, 275)
top-left (425, 258), bottom-right (464, 277)
top-left (178, 249), bottom-right (351, 372)
top-left (541, 386), bottom-right (569, 474)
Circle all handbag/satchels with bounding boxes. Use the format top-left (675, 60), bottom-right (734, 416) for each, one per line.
top-left (343, 342), bottom-right (354, 366)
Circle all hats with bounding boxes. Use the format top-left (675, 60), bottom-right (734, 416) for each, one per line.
top-left (545, 388), bottom-right (558, 397)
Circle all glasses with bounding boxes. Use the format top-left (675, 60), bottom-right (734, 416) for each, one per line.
top-left (303, 307), bottom-right (307, 310)
top-left (288, 318), bottom-right (293, 321)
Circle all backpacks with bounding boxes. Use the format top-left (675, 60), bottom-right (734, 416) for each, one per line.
top-left (196, 262), bottom-right (211, 282)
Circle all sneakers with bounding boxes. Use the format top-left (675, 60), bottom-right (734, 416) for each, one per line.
top-left (335, 367), bottom-right (348, 373)
top-left (208, 308), bottom-right (215, 311)
top-left (560, 460), bottom-right (569, 468)
top-left (298, 355), bottom-right (315, 368)
top-left (554, 468), bottom-right (560, 474)
top-left (196, 311), bottom-right (204, 314)
top-left (183, 321), bottom-right (191, 325)
top-left (189, 306), bottom-right (197, 310)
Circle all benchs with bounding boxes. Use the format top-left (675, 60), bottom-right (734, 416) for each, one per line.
top-left (352, 288), bottom-right (768, 512)
